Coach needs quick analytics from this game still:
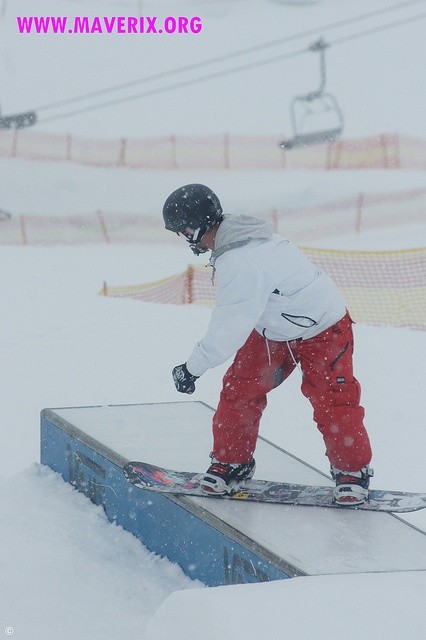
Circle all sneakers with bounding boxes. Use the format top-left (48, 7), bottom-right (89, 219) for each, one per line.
top-left (201, 451), bottom-right (256, 495)
top-left (330, 464), bottom-right (374, 507)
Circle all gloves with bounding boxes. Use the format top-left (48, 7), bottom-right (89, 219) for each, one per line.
top-left (172, 362), bottom-right (200, 395)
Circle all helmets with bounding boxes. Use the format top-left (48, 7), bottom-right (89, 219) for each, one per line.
top-left (162, 184), bottom-right (223, 256)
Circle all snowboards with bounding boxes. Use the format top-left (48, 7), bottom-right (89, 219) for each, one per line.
top-left (124, 461), bottom-right (425, 513)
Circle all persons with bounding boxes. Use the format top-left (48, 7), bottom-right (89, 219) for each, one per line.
top-left (163, 181), bottom-right (374, 507)
